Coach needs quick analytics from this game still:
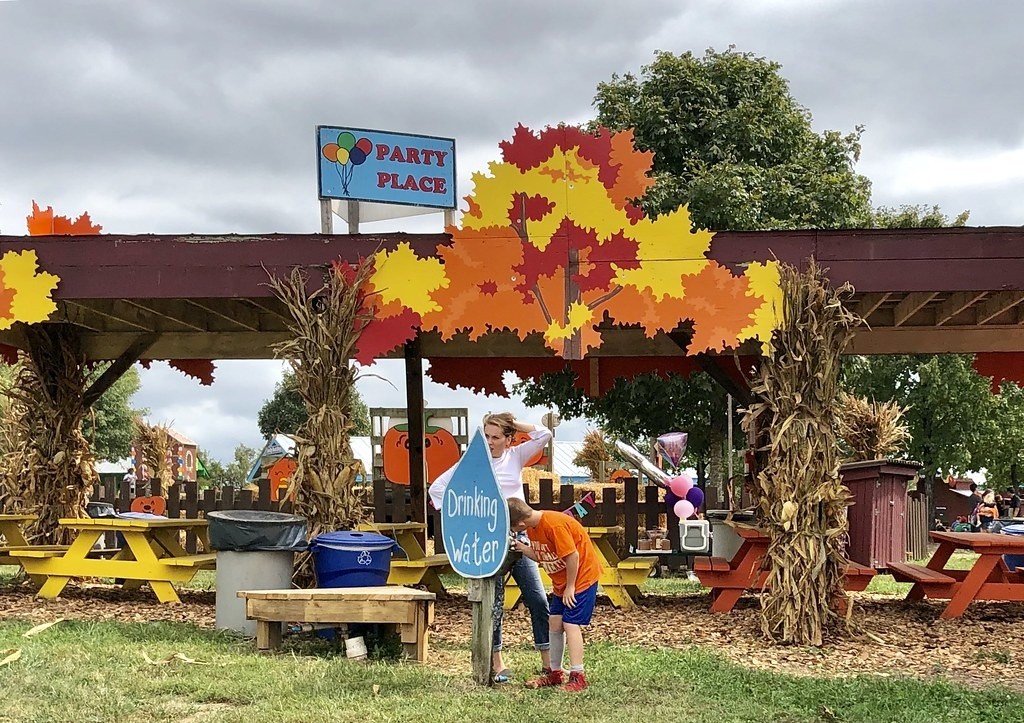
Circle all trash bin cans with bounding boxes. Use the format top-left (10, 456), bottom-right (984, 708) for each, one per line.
top-left (312, 530), bottom-right (394, 641)
top-left (1001, 524), bottom-right (1024, 571)
top-left (206, 510), bottom-right (308, 636)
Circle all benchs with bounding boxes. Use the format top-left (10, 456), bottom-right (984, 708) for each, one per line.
top-left (838, 559), bottom-right (877, 590)
top-left (388, 553), bottom-right (451, 586)
top-left (694, 556), bottom-right (730, 587)
top-left (503, 556), bottom-right (659, 583)
top-left (886, 561), bottom-right (957, 597)
top-left (0, 544), bottom-right (216, 604)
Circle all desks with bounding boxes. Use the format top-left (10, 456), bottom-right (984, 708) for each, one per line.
top-left (505, 526), bottom-right (644, 610)
top-left (358, 521), bottom-right (443, 596)
top-left (58, 517), bottom-right (210, 560)
top-left (0, 514), bottom-right (38, 545)
top-left (905, 531), bottom-right (1024, 618)
top-left (708, 529), bottom-right (848, 613)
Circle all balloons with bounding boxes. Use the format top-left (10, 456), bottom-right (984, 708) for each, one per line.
top-left (653, 432), bottom-right (703, 520)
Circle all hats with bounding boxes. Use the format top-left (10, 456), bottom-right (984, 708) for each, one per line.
top-left (960, 516), bottom-right (966, 523)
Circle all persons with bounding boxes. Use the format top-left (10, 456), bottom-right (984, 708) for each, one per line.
top-left (428, 413), bottom-right (553, 682)
top-left (122, 468), bottom-right (138, 492)
top-left (507, 497), bottom-right (604, 692)
top-left (936, 483), bottom-right (1021, 533)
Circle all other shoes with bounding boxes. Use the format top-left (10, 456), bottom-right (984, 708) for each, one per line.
top-left (560, 671), bottom-right (589, 692)
top-left (976, 522), bottom-right (981, 527)
top-left (525, 670), bottom-right (565, 688)
top-left (539, 667), bottom-right (552, 674)
top-left (490, 668), bottom-right (512, 683)
